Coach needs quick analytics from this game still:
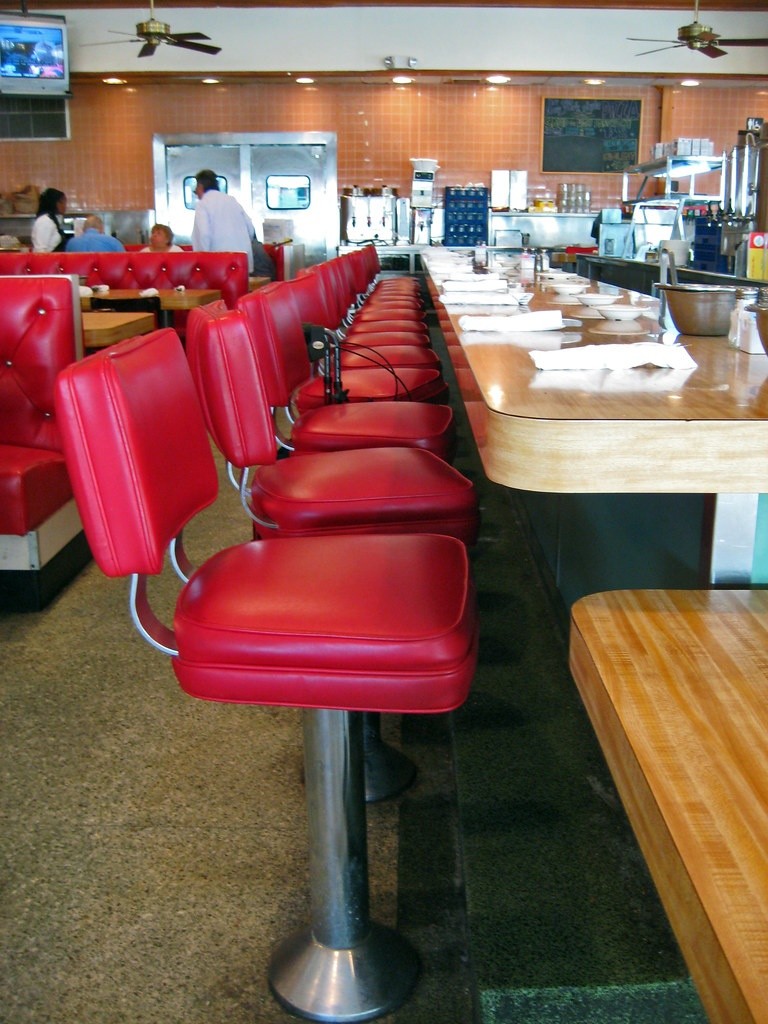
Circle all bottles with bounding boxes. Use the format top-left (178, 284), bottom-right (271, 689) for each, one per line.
top-left (730, 284), bottom-right (768, 355)
top-left (473, 239), bottom-right (488, 273)
top-left (521, 246), bottom-right (550, 272)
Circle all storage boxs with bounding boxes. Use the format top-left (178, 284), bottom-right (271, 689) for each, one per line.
top-left (444, 186), bottom-right (488, 249)
top-left (693, 216), bottom-right (728, 272)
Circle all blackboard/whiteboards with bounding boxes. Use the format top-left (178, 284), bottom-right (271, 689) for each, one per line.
top-left (538, 95), bottom-right (644, 177)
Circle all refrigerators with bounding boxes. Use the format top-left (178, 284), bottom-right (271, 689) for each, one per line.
top-left (151, 130), bottom-right (341, 268)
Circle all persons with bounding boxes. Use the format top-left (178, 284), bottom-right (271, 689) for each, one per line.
top-left (251, 235), bottom-right (274, 278)
top-left (31, 188), bottom-right (69, 253)
top-left (192, 169), bottom-right (255, 277)
top-left (139, 223), bottom-right (184, 252)
top-left (65, 215), bottom-right (126, 253)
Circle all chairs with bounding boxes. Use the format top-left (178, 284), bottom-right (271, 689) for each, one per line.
top-left (90, 297), bottom-right (162, 329)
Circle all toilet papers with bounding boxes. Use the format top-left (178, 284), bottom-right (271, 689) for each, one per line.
top-left (490, 170), bottom-right (509, 208)
top-left (510, 169), bottom-right (528, 211)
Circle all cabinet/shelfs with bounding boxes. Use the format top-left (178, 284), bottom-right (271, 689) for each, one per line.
top-left (338, 244), bottom-right (426, 273)
top-left (486, 212), bottom-right (598, 248)
top-left (624, 149), bottom-right (729, 264)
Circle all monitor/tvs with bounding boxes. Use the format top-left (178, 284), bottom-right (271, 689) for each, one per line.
top-left (0, 19), bottom-right (72, 97)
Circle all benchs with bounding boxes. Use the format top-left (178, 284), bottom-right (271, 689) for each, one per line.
top-left (0, 244), bottom-right (286, 612)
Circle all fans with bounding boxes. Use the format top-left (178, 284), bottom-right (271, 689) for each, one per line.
top-left (627, 0), bottom-right (768, 59)
top-left (79, 0), bottom-right (222, 58)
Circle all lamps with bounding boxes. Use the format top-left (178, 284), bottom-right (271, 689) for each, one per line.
top-left (385, 56), bottom-right (417, 69)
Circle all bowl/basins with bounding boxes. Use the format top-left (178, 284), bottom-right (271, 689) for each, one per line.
top-left (548, 294), bottom-right (586, 306)
top-left (652, 281), bottom-right (758, 336)
top-left (79, 275), bottom-right (88, 286)
top-left (498, 259), bottom-right (521, 268)
top-left (588, 316), bottom-right (651, 337)
top-left (537, 269), bottom-right (650, 319)
top-left (570, 305), bottom-right (605, 319)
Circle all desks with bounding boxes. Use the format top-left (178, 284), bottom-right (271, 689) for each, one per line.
top-left (424, 252), bottom-right (768, 589)
top-left (247, 275), bottom-right (270, 293)
top-left (80, 287), bottom-right (225, 328)
top-left (570, 589), bottom-right (768, 1024)
top-left (82, 314), bottom-right (155, 349)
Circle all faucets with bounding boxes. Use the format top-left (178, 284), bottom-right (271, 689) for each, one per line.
top-left (351, 216), bottom-right (356, 227)
top-left (420, 221), bottom-right (424, 231)
top-left (367, 217), bottom-right (371, 227)
top-left (382, 216), bottom-right (386, 227)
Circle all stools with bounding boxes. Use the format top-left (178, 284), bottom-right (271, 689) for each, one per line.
top-left (55, 244), bottom-right (478, 1021)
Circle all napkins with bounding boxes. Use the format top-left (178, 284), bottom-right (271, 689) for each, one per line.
top-left (528, 341), bottom-right (697, 372)
top-left (459, 310), bottom-right (563, 332)
top-left (424, 248), bottom-right (535, 305)
